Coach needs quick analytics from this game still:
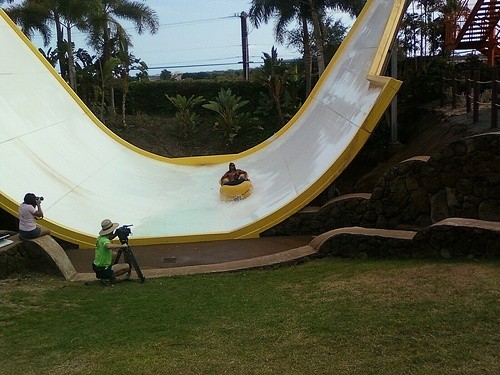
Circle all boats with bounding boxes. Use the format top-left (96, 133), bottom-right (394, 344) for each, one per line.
top-left (219, 169), bottom-right (252, 201)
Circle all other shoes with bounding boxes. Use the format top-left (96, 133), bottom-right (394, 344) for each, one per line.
top-left (102, 278), bottom-right (113, 285)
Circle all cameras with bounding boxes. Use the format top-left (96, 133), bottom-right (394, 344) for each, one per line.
top-left (36, 197), bottom-right (44, 201)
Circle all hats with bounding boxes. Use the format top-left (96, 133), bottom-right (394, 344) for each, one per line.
top-left (99, 219), bottom-right (119, 235)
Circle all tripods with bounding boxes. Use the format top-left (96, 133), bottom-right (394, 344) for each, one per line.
top-left (113, 239), bottom-right (146, 284)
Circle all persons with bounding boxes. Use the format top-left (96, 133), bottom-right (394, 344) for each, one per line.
top-left (92, 220), bottom-right (131, 286)
top-left (18, 194), bottom-right (50, 238)
top-left (220, 162), bottom-right (248, 185)
